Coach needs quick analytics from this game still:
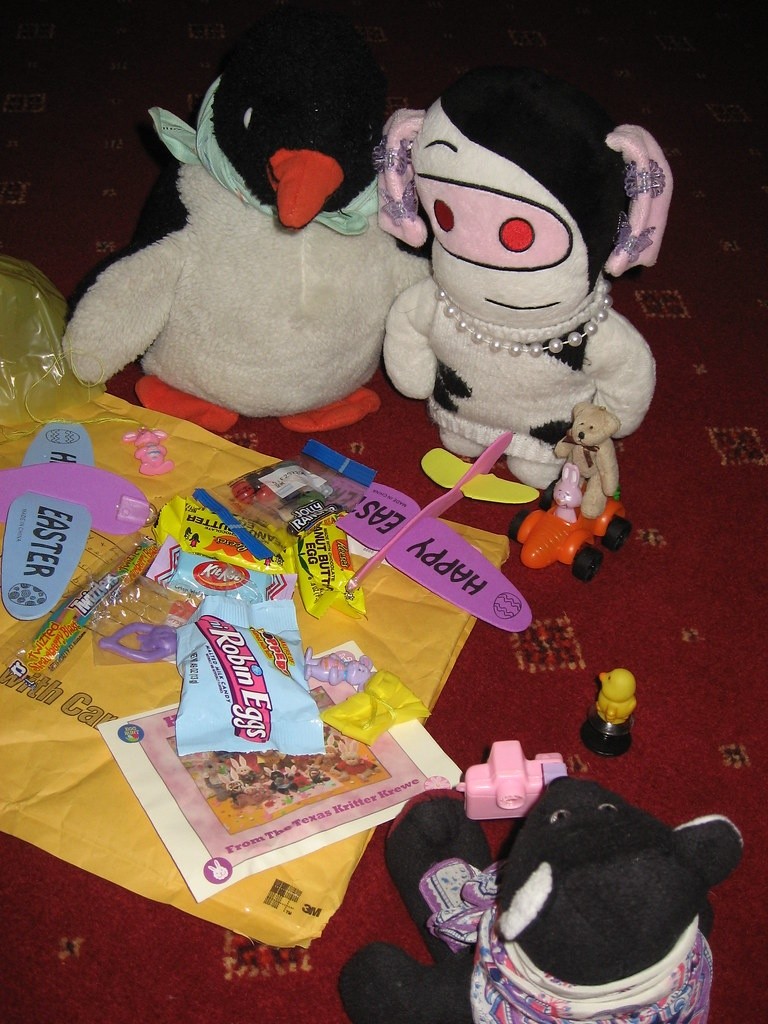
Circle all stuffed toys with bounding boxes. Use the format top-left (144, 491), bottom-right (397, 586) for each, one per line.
top-left (62, 16), bottom-right (677, 518)
top-left (336, 776), bottom-right (743, 1024)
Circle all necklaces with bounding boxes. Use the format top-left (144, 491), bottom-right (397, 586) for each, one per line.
top-left (435, 281), bottom-right (613, 358)
